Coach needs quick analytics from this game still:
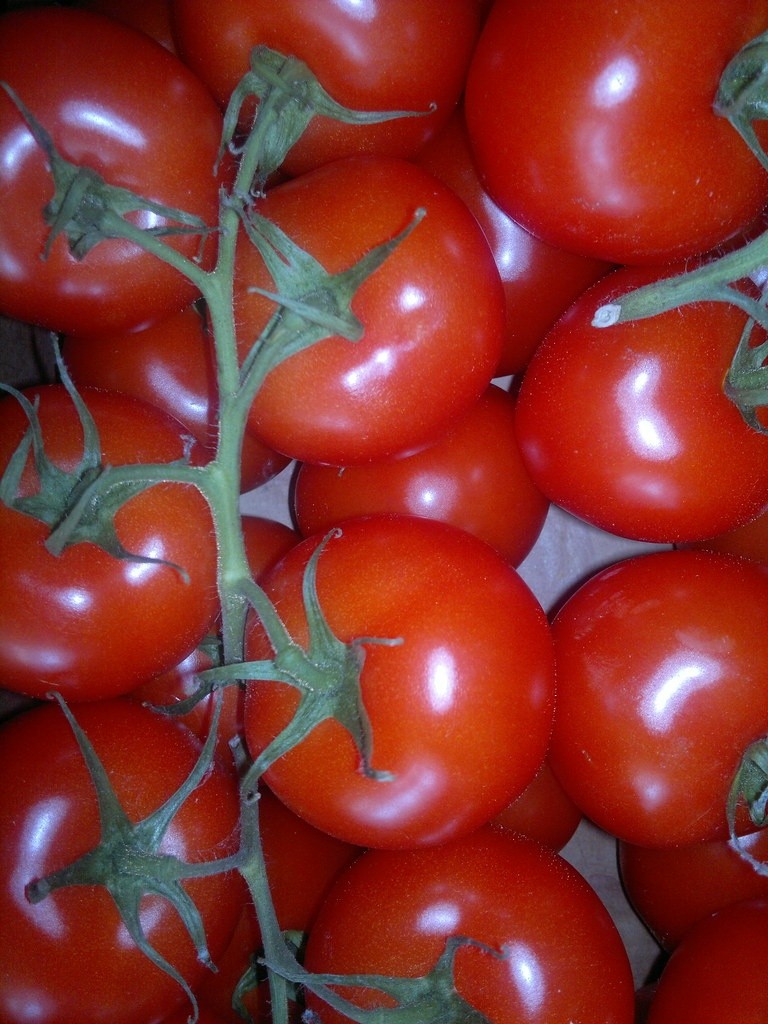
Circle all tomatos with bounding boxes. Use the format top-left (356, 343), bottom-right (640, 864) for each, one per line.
top-left (1, 0), bottom-right (768, 1024)
